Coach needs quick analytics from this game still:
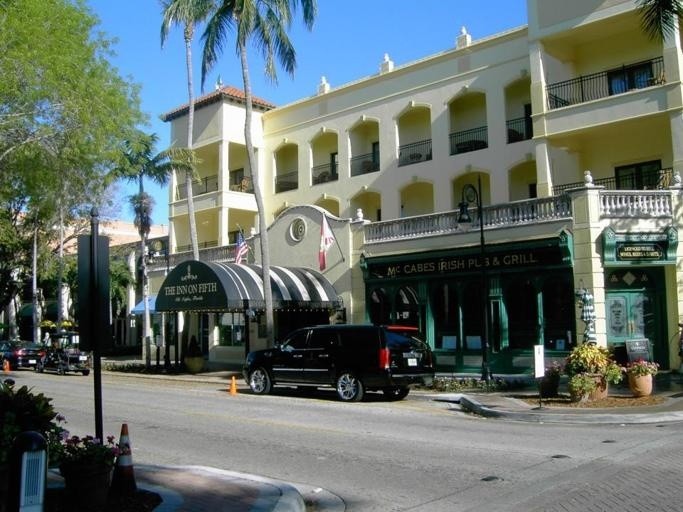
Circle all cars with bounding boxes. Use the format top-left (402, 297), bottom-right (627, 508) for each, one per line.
top-left (242, 323), bottom-right (435, 401)
top-left (0, 340), bottom-right (89, 375)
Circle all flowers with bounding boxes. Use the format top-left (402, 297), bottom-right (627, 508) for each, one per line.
top-left (530, 361), bottom-right (562, 382)
top-left (47, 415), bottom-right (130, 472)
top-left (624, 357), bottom-right (661, 376)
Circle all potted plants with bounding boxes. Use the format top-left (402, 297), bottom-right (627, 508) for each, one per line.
top-left (183, 343), bottom-right (206, 373)
top-left (563, 340), bottom-right (624, 404)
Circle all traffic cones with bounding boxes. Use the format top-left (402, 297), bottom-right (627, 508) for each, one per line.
top-left (229, 376), bottom-right (237, 396)
top-left (110, 424), bottom-right (136, 496)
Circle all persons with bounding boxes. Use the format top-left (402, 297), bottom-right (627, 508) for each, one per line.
top-left (43, 332), bottom-right (53, 347)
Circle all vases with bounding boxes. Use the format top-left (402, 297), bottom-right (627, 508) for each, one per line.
top-left (628, 372), bottom-right (653, 397)
top-left (64, 468), bottom-right (111, 512)
top-left (537, 376), bottom-right (559, 398)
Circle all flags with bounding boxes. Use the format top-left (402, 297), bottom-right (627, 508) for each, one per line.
top-left (233, 231), bottom-right (250, 265)
top-left (319, 212), bottom-right (335, 271)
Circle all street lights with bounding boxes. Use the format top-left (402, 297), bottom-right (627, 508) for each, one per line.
top-left (457, 175), bottom-right (491, 380)
